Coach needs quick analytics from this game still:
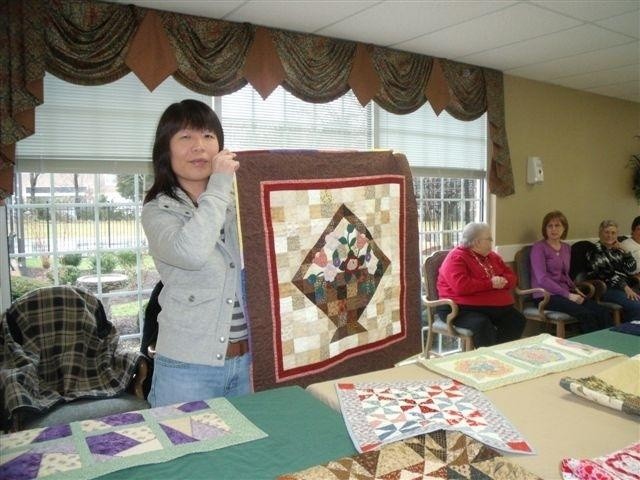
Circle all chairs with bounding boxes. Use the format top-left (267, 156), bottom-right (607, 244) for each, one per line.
top-left (514, 245), bottom-right (595, 340)
top-left (0, 286), bottom-right (148, 433)
top-left (424, 250), bottom-right (501, 359)
top-left (571, 241), bottom-right (640, 327)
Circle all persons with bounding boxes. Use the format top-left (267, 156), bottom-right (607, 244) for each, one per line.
top-left (436, 220), bottom-right (527, 350)
top-left (619, 215), bottom-right (640, 284)
top-left (138, 98), bottom-right (254, 410)
top-left (580, 219), bottom-right (640, 322)
top-left (528, 210), bottom-right (614, 336)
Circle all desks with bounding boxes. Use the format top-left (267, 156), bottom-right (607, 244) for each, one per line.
top-left (0, 385), bottom-right (359, 480)
top-left (305, 322), bottom-right (639, 479)
